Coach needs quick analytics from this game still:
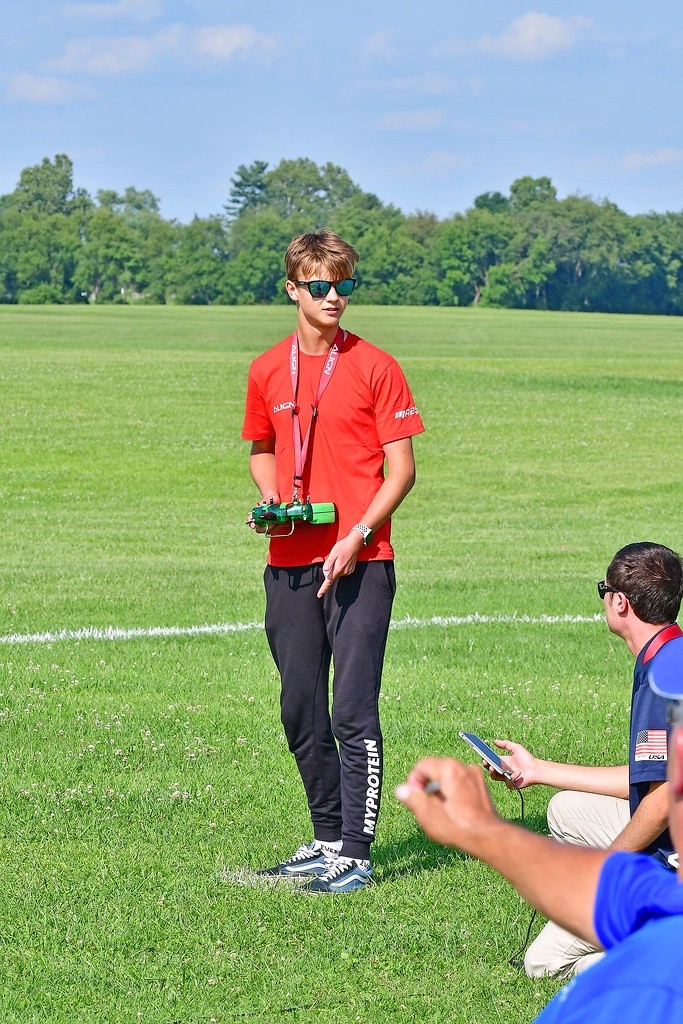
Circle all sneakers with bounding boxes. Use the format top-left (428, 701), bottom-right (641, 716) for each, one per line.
top-left (295, 855), bottom-right (376, 895)
top-left (256, 838), bottom-right (343, 878)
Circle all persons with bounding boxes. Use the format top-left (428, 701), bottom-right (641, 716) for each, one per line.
top-left (242, 228), bottom-right (424, 894)
top-left (484, 543), bottom-right (683, 983)
top-left (393, 698), bottom-right (683, 1024)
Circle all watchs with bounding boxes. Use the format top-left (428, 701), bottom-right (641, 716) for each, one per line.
top-left (351, 524), bottom-right (374, 546)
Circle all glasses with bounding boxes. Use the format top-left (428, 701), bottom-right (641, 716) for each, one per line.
top-left (598, 580), bottom-right (631, 599)
top-left (293, 278), bottom-right (356, 298)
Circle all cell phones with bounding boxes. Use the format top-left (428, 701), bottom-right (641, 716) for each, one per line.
top-left (458, 732), bottom-right (514, 776)
